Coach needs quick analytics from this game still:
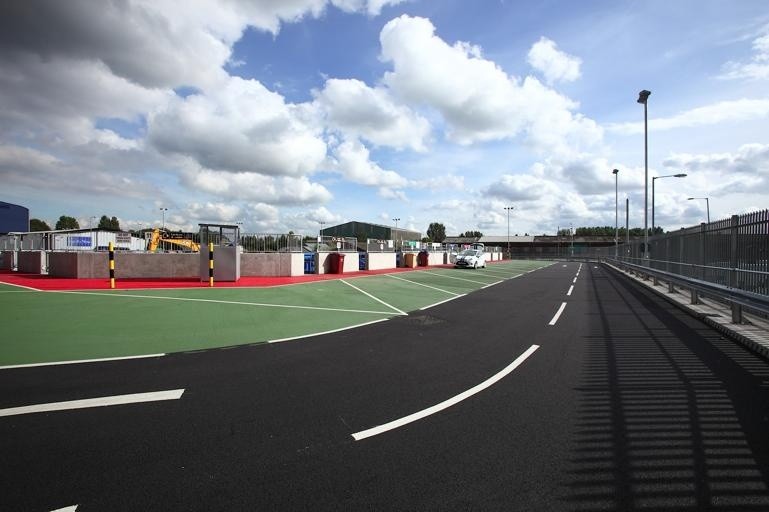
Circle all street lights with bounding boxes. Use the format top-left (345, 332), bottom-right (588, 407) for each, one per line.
top-left (688, 197), bottom-right (710, 225)
top-left (504, 207), bottom-right (514, 261)
top-left (392, 218), bottom-right (401, 253)
top-left (652, 174), bottom-right (687, 236)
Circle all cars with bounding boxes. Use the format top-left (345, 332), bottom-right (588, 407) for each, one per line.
top-left (453, 249), bottom-right (487, 270)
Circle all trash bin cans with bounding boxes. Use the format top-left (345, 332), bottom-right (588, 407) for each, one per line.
top-left (405, 254), bottom-right (416, 268)
top-left (419, 252), bottom-right (429, 266)
top-left (330, 253), bottom-right (346, 274)
